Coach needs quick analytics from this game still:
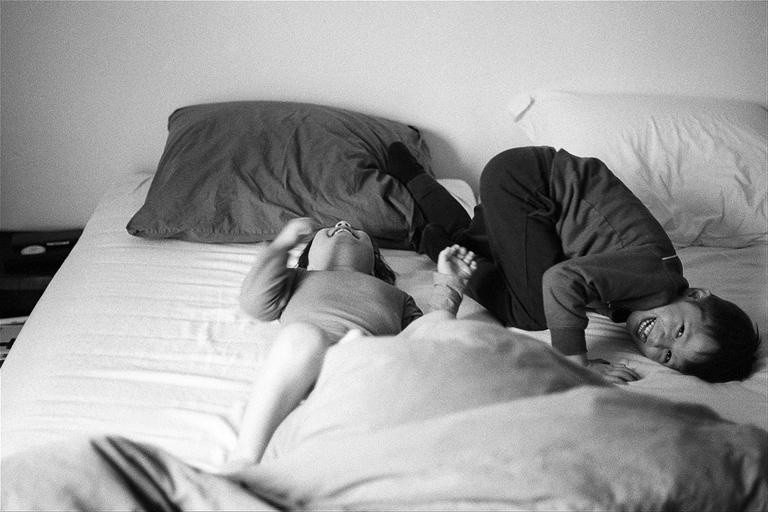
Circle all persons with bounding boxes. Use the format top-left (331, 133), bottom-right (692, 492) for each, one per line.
top-left (385, 141), bottom-right (762, 388)
top-left (227, 217), bottom-right (477, 465)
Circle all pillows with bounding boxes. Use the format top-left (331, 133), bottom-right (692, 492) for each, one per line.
top-left (506, 92), bottom-right (766, 249)
top-left (125, 100), bottom-right (441, 250)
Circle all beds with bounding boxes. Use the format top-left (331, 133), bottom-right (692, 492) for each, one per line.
top-left (1, 153), bottom-right (765, 510)
